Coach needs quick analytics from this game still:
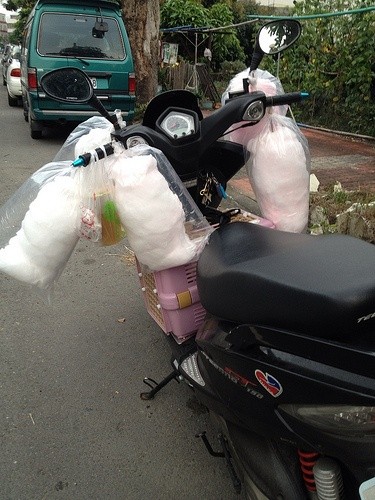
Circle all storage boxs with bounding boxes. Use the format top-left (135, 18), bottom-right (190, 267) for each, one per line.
top-left (134, 208), bottom-right (276, 339)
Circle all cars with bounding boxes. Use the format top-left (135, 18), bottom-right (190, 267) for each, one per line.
top-left (1, 44), bottom-right (23, 106)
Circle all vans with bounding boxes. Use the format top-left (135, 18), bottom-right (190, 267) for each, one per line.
top-left (20, 0), bottom-right (138, 139)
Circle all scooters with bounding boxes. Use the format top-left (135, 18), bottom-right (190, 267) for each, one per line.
top-left (38, 18), bottom-right (375, 500)
